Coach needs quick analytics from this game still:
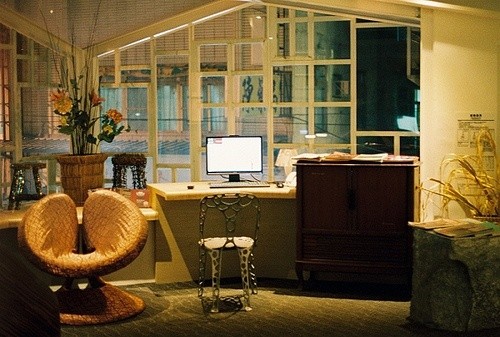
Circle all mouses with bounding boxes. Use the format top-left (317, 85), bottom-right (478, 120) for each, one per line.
top-left (277, 182), bottom-right (283, 188)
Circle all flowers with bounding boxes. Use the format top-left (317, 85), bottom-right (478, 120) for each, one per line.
top-left (38, 1), bottom-right (126, 154)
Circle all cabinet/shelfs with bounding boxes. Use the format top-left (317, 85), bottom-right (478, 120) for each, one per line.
top-left (293, 160), bottom-right (420, 287)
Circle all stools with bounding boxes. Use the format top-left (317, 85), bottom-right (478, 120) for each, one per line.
top-left (111, 151), bottom-right (147, 193)
top-left (8, 162), bottom-right (47, 211)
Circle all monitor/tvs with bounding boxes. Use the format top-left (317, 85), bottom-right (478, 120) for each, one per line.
top-left (205, 135), bottom-right (263, 181)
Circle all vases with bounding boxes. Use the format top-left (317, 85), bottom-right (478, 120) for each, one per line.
top-left (57, 153), bottom-right (107, 206)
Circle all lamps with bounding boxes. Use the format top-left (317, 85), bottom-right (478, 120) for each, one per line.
top-left (274, 149), bottom-right (300, 178)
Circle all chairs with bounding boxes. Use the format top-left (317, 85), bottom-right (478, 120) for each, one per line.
top-left (197, 193), bottom-right (263, 313)
top-left (19, 190), bottom-right (145, 326)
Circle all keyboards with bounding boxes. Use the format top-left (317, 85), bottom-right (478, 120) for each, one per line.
top-left (209, 182), bottom-right (271, 188)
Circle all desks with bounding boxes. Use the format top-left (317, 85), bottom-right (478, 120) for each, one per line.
top-left (145, 184), bottom-right (295, 288)
top-left (0, 198), bottom-right (157, 282)
